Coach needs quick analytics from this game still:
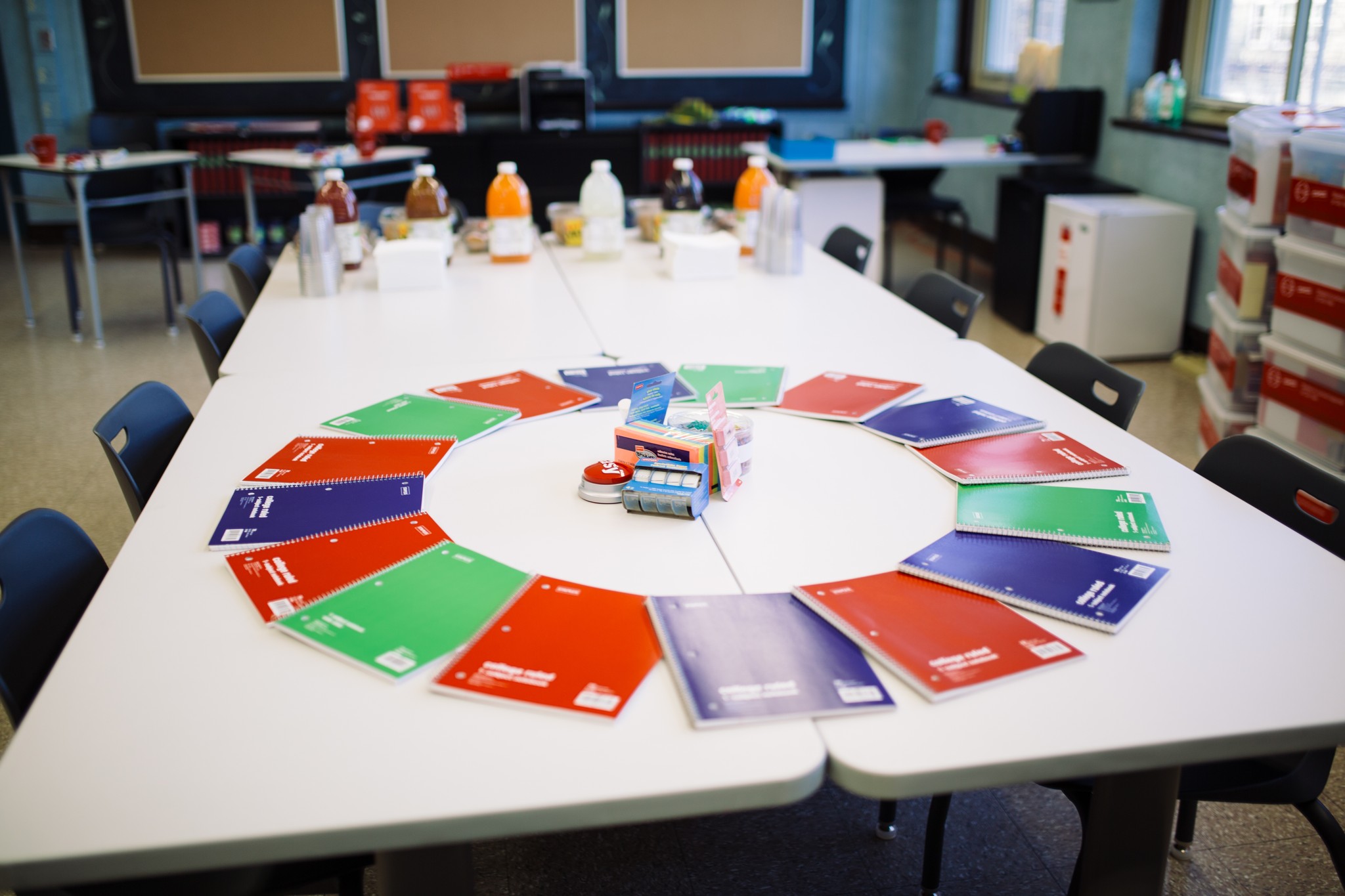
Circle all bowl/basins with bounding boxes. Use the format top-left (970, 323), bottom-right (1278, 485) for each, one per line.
top-left (631, 196), bottom-right (710, 243)
top-left (378, 204), bottom-right (457, 240)
top-left (544, 198), bottom-right (586, 247)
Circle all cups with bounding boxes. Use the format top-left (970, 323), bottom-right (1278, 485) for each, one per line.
top-left (925, 118), bottom-right (948, 143)
top-left (297, 200), bottom-right (342, 300)
top-left (26, 133), bottom-right (57, 164)
top-left (753, 184), bottom-right (804, 275)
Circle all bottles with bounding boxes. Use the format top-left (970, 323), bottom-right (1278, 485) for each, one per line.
top-left (315, 167), bottom-right (361, 268)
top-left (733, 155), bottom-right (778, 255)
top-left (405, 161), bottom-right (454, 270)
top-left (485, 159), bottom-right (536, 264)
top-left (664, 158), bottom-right (705, 213)
top-left (579, 159), bottom-right (626, 265)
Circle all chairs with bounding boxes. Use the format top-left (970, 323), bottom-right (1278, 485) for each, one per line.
top-left (188, 290), bottom-right (245, 385)
top-left (820, 223), bottom-right (873, 277)
top-left (859, 120), bottom-right (975, 294)
top-left (1020, 342), bottom-right (1147, 433)
top-left (877, 432), bottom-right (1344, 896)
top-left (228, 241), bottom-right (270, 309)
top-left (0, 508), bottom-right (112, 737)
top-left (92, 381), bottom-right (195, 521)
top-left (902, 268), bottom-right (987, 341)
top-left (58, 112), bottom-right (186, 345)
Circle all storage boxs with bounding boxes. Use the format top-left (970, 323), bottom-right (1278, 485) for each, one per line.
top-left (1194, 108), bottom-right (1344, 492)
top-left (614, 418), bottom-right (722, 494)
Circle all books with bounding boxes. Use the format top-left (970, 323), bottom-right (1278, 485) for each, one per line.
top-left (205, 470), bottom-right (427, 551)
top-left (641, 130), bottom-right (771, 186)
top-left (904, 429), bottom-right (1130, 487)
top-left (427, 370), bottom-right (600, 428)
top-left (854, 392), bottom-right (1050, 449)
top-left (669, 361), bottom-right (786, 411)
top-left (223, 507), bottom-right (456, 626)
top-left (898, 524), bottom-right (1169, 636)
top-left (794, 569), bottom-right (1084, 704)
top-left (953, 475), bottom-right (1170, 552)
top-left (644, 591), bottom-right (897, 733)
top-left (428, 573), bottom-right (663, 722)
top-left (755, 370), bottom-right (924, 423)
top-left (558, 363), bottom-right (697, 415)
top-left (321, 390), bottom-right (522, 449)
top-left (266, 537), bottom-right (535, 683)
top-left (239, 432), bottom-right (459, 485)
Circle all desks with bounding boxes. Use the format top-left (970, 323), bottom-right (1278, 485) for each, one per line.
top-left (218, 213), bottom-right (607, 374)
top-left (544, 223), bottom-right (955, 353)
top-left (0, 375), bottom-right (827, 892)
top-left (630, 352), bottom-right (1345, 896)
top-left (229, 144), bottom-right (429, 246)
top-left (1, 147), bottom-right (203, 350)
top-left (744, 131), bottom-right (1087, 185)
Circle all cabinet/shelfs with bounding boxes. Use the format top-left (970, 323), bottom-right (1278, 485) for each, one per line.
top-left (146, 111), bottom-right (787, 259)
